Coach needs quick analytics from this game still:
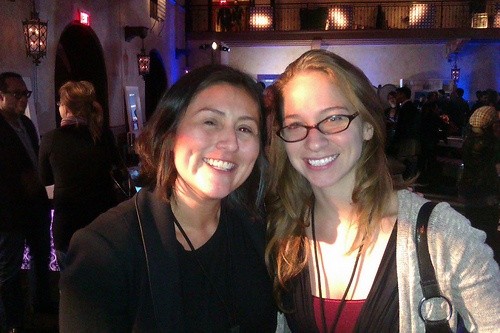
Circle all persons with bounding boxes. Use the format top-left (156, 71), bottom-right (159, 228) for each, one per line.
top-left (0, 72), bottom-right (59, 333)
top-left (231, 0), bottom-right (244, 32)
top-left (263, 50), bottom-right (500, 333)
top-left (59, 63), bottom-right (278, 333)
top-left (456, 105), bottom-right (500, 231)
top-left (217, 2), bottom-right (231, 32)
top-left (384, 86), bottom-right (420, 179)
top-left (415, 88), bottom-right (500, 136)
top-left (38, 80), bottom-right (116, 268)
top-left (256, 81), bottom-right (265, 93)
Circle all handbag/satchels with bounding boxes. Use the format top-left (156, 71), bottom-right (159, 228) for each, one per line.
top-left (108, 165), bottom-right (137, 207)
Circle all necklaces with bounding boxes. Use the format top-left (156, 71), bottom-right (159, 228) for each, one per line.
top-left (311, 194), bottom-right (378, 333)
top-left (170, 201), bottom-right (240, 333)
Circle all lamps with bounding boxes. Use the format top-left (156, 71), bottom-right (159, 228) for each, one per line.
top-left (22, 0), bottom-right (48, 66)
top-left (125, 26), bottom-right (151, 78)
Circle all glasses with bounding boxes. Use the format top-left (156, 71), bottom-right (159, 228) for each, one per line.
top-left (276, 112), bottom-right (360, 143)
top-left (57, 102), bottom-right (65, 105)
top-left (0, 91), bottom-right (32, 99)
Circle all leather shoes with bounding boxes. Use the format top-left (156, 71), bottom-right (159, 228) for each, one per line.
top-left (7, 320), bottom-right (20, 333)
